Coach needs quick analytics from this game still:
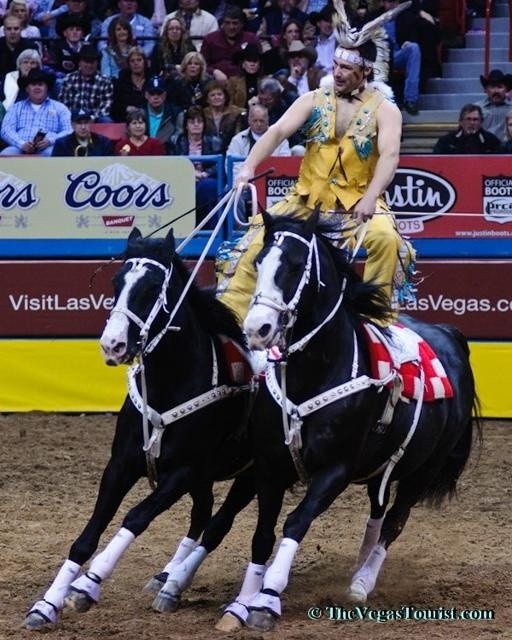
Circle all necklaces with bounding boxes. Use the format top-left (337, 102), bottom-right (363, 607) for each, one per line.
top-left (333, 82), bottom-right (369, 102)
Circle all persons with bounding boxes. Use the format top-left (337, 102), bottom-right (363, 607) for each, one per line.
top-left (167, 109), bottom-right (223, 156)
top-left (200, 5), bottom-right (261, 84)
top-left (225, 104), bottom-right (292, 157)
top-left (224, 40), bottom-right (268, 106)
top-left (97, 17), bottom-right (136, 80)
top-left (414, 0), bottom-right (438, 47)
top-left (111, 47), bottom-right (159, 121)
top-left (0, 14), bottom-right (37, 79)
top-left (216, 35), bottom-right (403, 331)
top-left (200, 80), bottom-right (243, 134)
top-left (32, 0), bottom-right (68, 39)
top-left (137, 74), bottom-right (183, 142)
top-left (0, 69), bottom-right (73, 157)
top-left (201, 1), bottom-right (260, 30)
top-left (0, 1), bottom-right (41, 38)
top-left (271, 44), bottom-right (327, 104)
top-left (433, 105), bottom-right (503, 154)
top-left (503, 111), bottom-right (512, 155)
top-left (472, 69), bottom-right (512, 142)
top-left (47, 1), bottom-right (101, 42)
top-left (3, 49), bottom-right (43, 112)
top-left (113, 109), bottom-right (166, 155)
top-left (44, 10), bottom-right (91, 74)
top-left (55, 45), bottom-right (114, 123)
top-left (148, 15), bottom-right (197, 77)
top-left (347, 0), bottom-right (421, 114)
top-left (136, 0), bottom-right (180, 30)
top-left (95, 6), bottom-right (113, 20)
top-left (304, 4), bottom-right (341, 74)
top-left (51, 108), bottom-right (113, 157)
top-left (173, 51), bottom-right (215, 108)
top-left (261, 19), bottom-right (303, 76)
top-left (228, 79), bottom-right (303, 145)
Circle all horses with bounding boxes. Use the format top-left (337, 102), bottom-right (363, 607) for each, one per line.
top-left (24, 226), bottom-right (263, 629)
top-left (242, 201), bottom-right (483, 633)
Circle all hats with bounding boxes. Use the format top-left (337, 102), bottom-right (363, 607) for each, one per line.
top-left (479, 70), bottom-right (512, 94)
top-left (18, 70), bottom-right (55, 88)
top-left (147, 76), bottom-right (168, 92)
top-left (55, 11), bottom-right (91, 36)
top-left (307, 7), bottom-right (337, 26)
top-left (239, 44), bottom-right (261, 67)
top-left (281, 39), bottom-right (318, 68)
top-left (71, 109), bottom-right (91, 122)
top-left (77, 45), bottom-right (103, 61)
